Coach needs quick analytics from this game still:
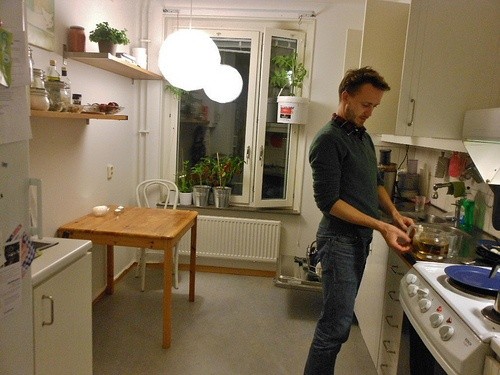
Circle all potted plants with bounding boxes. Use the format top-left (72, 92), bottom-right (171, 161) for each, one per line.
top-left (89, 21), bottom-right (130, 56)
top-left (190, 157), bottom-right (214, 207)
top-left (271, 50), bottom-right (309, 125)
top-left (174, 160), bottom-right (192, 206)
top-left (211, 152), bottom-right (246, 208)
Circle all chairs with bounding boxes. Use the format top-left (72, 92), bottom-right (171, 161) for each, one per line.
top-left (134, 179), bottom-right (180, 292)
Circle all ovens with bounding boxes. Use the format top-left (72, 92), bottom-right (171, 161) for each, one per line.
top-left (392, 266), bottom-right (497, 375)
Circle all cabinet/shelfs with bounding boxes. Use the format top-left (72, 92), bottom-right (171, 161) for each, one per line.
top-left (343, 0), bottom-right (500, 152)
top-left (353, 231), bottom-right (409, 375)
top-left (27, 236), bottom-right (92, 375)
top-left (30, 50), bottom-right (163, 120)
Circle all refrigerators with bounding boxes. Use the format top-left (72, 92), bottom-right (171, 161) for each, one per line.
top-left (1, 1), bottom-right (37, 375)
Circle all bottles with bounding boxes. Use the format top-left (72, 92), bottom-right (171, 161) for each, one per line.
top-left (114, 206), bottom-right (124, 219)
top-left (68, 26), bottom-right (86, 52)
top-left (27, 47), bottom-right (82, 113)
top-left (448, 152), bottom-right (463, 177)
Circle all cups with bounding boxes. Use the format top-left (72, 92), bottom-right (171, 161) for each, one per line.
top-left (407, 159), bottom-right (418, 174)
top-left (380, 148), bottom-right (392, 165)
top-left (446, 232), bottom-right (458, 258)
top-left (132, 47), bottom-right (148, 69)
top-left (415, 195), bottom-right (426, 211)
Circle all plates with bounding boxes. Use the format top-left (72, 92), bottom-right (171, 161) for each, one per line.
top-left (476, 240), bottom-right (500, 267)
top-left (444, 265), bottom-right (500, 296)
top-left (82, 105), bottom-right (126, 115)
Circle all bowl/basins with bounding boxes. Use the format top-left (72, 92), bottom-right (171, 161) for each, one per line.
top-left (93, 206), bottom-right (107, 216)
top-left (410, 228), bottom-right (448, 261)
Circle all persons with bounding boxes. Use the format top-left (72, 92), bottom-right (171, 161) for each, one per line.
top-left (304, 67), bottom-right (415, 375)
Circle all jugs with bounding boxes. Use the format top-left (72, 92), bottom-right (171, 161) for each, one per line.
top-left (376, 163), bottom-right (398, 203)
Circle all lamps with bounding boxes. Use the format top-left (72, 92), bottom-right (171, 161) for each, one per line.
top-left (157, 0), bottom-right (243, 104)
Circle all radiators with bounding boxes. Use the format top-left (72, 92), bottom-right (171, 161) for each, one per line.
top-left (148, 216), bottom-right (282, 262)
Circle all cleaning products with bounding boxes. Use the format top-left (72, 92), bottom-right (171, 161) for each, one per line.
top-left (457, 186), bottom-right (476, 257)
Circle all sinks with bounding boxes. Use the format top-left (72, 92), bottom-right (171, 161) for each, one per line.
top-left (432, 225), bottom-right (474, 257)
top-left (403, 212), bottom-right (448, 224)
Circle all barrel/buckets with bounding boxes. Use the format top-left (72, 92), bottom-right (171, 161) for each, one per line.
top-left (397, 172), bottom-right (420, 199)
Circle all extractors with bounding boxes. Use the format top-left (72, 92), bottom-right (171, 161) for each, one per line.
top-left (461, 108), bottom-right (500, 143)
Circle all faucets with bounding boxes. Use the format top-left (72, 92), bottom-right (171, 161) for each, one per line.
top-left (432, 182), bottom-right (452, 199)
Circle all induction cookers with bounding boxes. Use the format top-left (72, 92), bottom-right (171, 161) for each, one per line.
top-left (411, 262), bottom-right (499, 345)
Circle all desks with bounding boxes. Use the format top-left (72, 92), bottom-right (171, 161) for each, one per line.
top-left (58, 203), bottom-right (198, 349)
